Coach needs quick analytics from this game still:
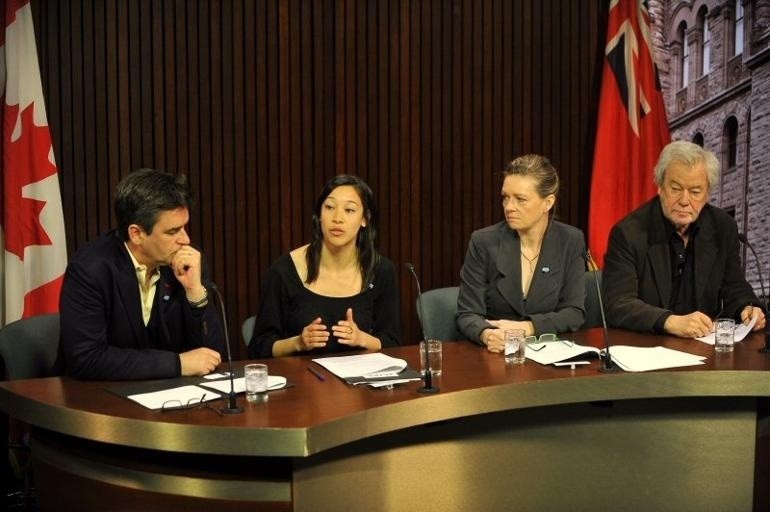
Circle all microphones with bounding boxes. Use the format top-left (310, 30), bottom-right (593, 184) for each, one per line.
top-left (208, 280), bottom-right (245, 414)
top-left (407, 262), bottom-right (440, 394)
top-left (737, 233), bottom-right (770, 353)
top-left (581, 251), bottom-right (618, 373)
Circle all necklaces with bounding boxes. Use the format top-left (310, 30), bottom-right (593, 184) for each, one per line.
top-left (520, 249), bottom-right (540, 273)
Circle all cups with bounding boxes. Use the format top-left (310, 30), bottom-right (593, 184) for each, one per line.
top-left (503, 329), bottom-right (526, 366)
top-left (714, 317), bottom-right (736, 353)
top-left (244, 363), bottom-right (269, 406)
top-left (418, 339), bottom-right (443, 378)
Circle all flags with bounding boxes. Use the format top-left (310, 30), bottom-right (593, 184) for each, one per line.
top-left (588, 0), bottom-right (678, 278)
top-left (0, 0), bottom-right (69, 334)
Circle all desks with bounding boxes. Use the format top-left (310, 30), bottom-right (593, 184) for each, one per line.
top-left (2, 321), bottom-right (770, 511)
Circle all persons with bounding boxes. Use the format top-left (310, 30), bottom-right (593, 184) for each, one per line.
top-left (598, 138), bottom-right (768, 337)
top-left (452, 154), bottom-right (589, 355)
top-left (49, 169), bottom-right (231, 380)
top-left (242, 173), bottom-right (401, 362)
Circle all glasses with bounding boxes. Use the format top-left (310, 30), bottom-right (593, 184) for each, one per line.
top-left (163, 395), bottom-right (207, 412)
top-left (524, 334), bottom-right (576, 351)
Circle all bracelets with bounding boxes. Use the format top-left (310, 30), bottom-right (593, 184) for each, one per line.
top-left (181, 287), bottom-right (211, 307)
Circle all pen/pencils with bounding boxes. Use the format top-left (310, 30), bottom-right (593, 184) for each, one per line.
top-left (308, 367), bottom-right (325, 381)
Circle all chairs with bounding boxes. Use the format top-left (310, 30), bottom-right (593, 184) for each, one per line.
top-left (1, 309), bottom-right (65, 381)
top-left (584, 269), bottom-right (608, 330)
top-left (240, 314), bottom-right (257, 348)
top-left (413, 284), bottom-right (459, 346)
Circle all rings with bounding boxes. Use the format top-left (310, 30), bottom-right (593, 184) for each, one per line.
top-left (348, 326), bottom-right (353, 334)
